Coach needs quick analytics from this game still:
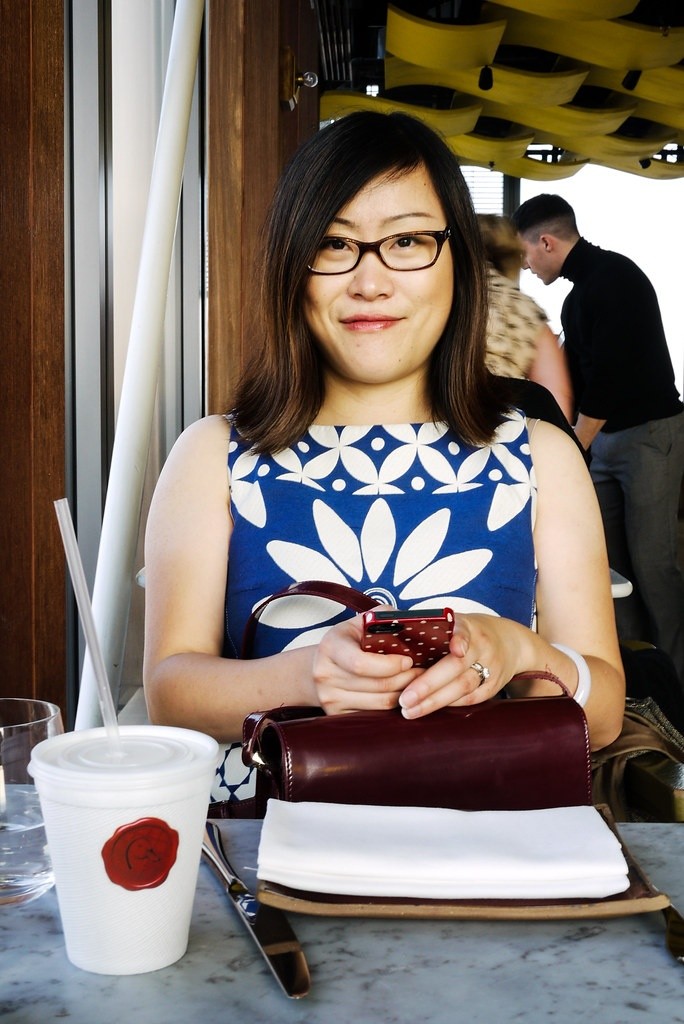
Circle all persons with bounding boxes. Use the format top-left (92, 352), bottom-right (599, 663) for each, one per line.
top-left (477, 194), bottom-right (683, 651)
top-left (143, 109), bottom-right (625, 819)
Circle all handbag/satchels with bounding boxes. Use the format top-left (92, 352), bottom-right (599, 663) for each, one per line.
top-left (257, 694), bottom-right (593, 813)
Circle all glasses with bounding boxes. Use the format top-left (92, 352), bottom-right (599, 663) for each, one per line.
top-left (309, 223), bottom-right (452, 275)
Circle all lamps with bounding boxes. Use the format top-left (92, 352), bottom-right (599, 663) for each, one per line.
top-left (622, 69), bottom-right (642, 92)
top-left (478, 65), bottom-right (494, 91)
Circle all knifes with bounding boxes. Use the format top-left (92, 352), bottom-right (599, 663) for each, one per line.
top-left (202, 822), bottom-right (312, 1000)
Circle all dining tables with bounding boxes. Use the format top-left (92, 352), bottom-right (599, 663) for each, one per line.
top-left (0, 781), bottom-right (684, 1024)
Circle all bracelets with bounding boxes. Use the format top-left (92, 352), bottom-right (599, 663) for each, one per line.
top-left (550, 643), bottom-right (591, 709)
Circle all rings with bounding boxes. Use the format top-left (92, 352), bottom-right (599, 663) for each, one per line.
top-left (470, 662), bottom-right (490, 687)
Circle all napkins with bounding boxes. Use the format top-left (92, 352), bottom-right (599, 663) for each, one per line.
top-left (253, 795), bottom-right (633, 900)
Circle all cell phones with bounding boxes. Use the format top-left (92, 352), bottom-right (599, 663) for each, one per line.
top-left (361, 607), bottom-right (454, 668)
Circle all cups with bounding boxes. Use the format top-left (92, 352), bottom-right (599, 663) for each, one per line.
top-left (0, 696), bottom-right (65, 909)
top-left (26, 724), bottom-right (221, 976)
